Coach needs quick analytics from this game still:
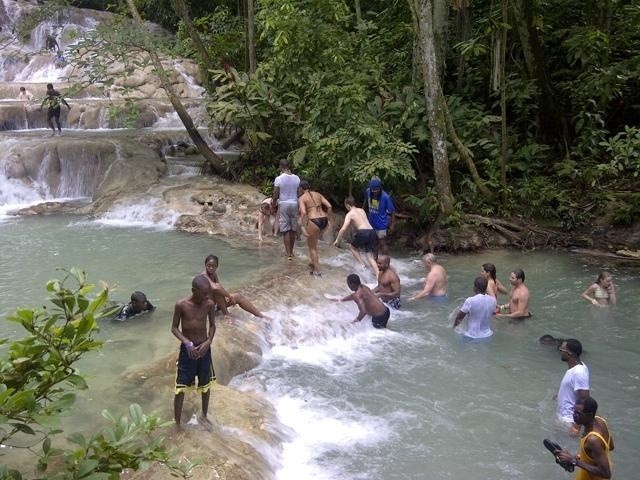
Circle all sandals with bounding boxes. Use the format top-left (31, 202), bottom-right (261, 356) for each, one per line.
top-left (310, 271), bottom-right (322, 277)
top-left (288, 255), bottom-right (293, 260)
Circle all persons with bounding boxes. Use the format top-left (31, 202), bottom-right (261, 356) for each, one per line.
top-left (370, 254), bottom-right (400, 309)
top-left (39, 84), bottom-right (71, 137)
top-left (219, 55), bottom-right (235, 80)
top-left (539, 335), bottom-right (563, 348)
top-left (454, 275), bottom-right (496, 338)
top-left (362, 175), bottom-right (394, 256)
top-left (409, 254), bottom-right (450, 306)
top-left (170, 275), bottom-right (216, 435)
top-left (17, 87), bottom-right (35, 102)
top-left (296, 180), bottom-right (331, 275)
top-left (326, 274), bottom-right (390, 328)
top-left (580, 272), bottom-right (617, 308)
top-left (53, 50), bottom-right (66, 67)
top-left (199, 254), bottom-right (271, 324)
top-left (334, 197), bottom-right (380, 279)
top-left (480, 262), bottom-right (533, 322)
top-left (255, 158), bottom-right (301, 259)
top-left (101, 291), bottom-right (158, 322)
top-left (554, 338), bottom-right (591, 439)
top-left (44, 34), bottom-right (61, 53)
top-left (553, 394), bottom-right (615, 480)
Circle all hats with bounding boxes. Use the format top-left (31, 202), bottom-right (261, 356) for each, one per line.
top-left (370, 176), bottom-right (383, 192)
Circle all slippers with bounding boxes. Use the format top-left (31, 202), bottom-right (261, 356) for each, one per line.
top-left (543, 439), bottom-right (575, 473)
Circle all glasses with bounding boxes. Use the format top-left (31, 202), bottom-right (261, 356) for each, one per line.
top-left (558, 346), bottom-right (571, 353)
top-left (572, 409), bottom-right (593, 418)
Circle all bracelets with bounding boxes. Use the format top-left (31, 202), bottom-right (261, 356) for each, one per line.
top-left (571, 457), bottom-right (578, 467)
top-left (591, 299), bottom-right (598, 305)
top-left (183, 341), bottom-right (194, 349)
top-left (228, 295), bottom-right (232, 299)
